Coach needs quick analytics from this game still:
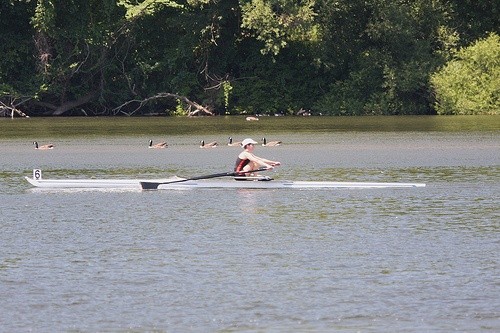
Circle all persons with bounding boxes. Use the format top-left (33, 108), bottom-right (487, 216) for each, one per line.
top-left (234, 138), bottom-right (279, 180)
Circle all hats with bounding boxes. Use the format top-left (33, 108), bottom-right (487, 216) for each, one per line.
top-left (241, 138), bottom-right (258, 148)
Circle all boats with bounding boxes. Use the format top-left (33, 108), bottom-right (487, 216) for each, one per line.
top-left (22, 167), bottom-right (428, 190)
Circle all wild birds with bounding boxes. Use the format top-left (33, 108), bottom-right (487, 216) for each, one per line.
top-left (200, 139), bottom-right (217, 149)
top-left (33, 141), bottom-right (55, 150)
top-left (228, 136), bottom-right (243, 146)
top-left (260, 136), bottom-right (282, 147)
top-left (149, 139), bottom-right (169, 149)
